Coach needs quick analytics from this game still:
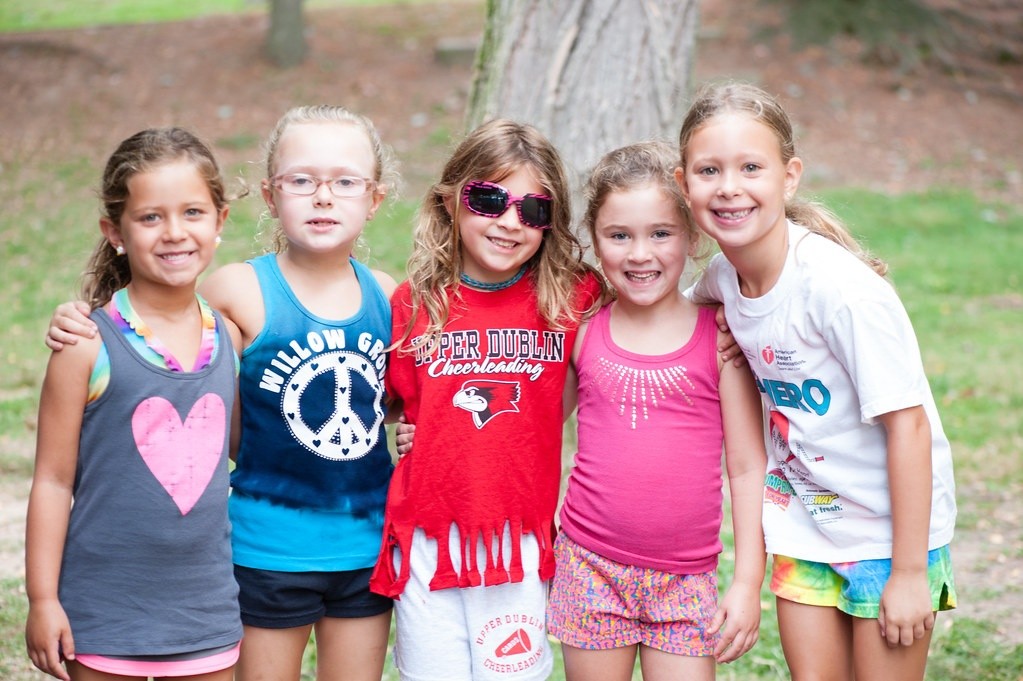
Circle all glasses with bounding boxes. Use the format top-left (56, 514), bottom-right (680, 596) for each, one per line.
top-left (459, 180), bottom-right (554, 229)
top-left (270, 173), bottom-right (378, 197)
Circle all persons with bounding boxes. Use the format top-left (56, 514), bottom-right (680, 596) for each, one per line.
top-left (44, 104), bottom-right (403, 681)
top-left (396, 137), bottom-right (768, 681)
top-left (384, 116), bottom-right (749, 681)
top-left (674, 82), bottom-right (959, 681)
top-left (26, 126), bottom-right (246, 681)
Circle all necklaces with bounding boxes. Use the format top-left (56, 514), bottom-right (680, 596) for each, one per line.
top-left (458, 262), bottom-right (529, 290)
